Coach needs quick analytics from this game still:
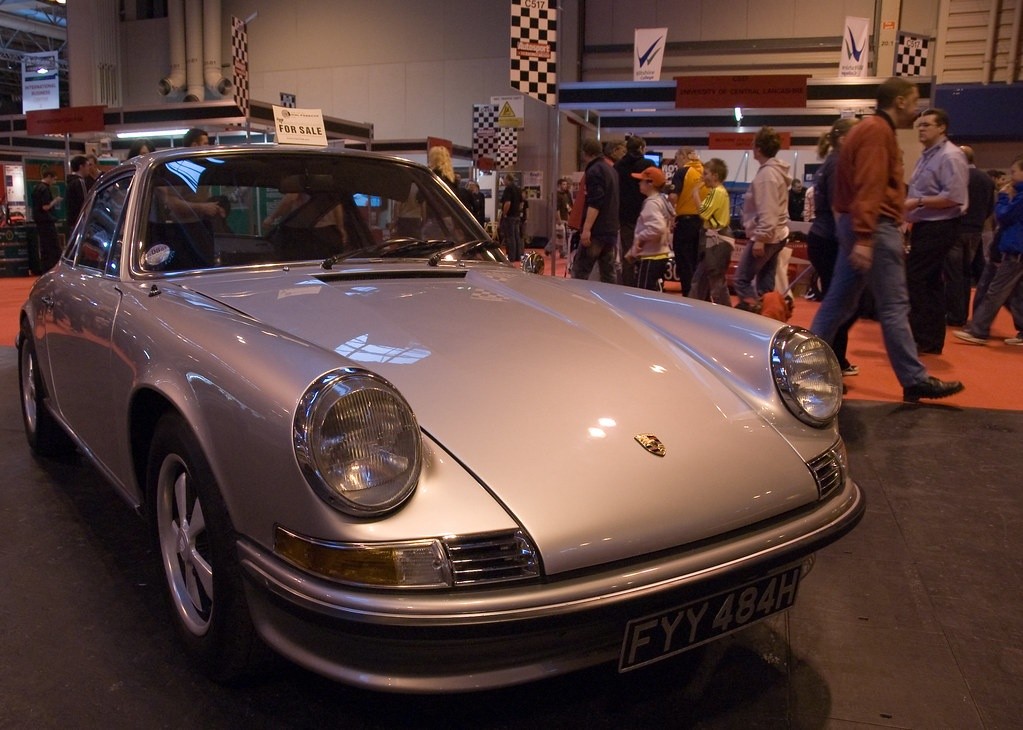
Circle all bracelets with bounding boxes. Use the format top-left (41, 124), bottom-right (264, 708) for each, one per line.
top-left (918, 197), bottom-right (923, 207)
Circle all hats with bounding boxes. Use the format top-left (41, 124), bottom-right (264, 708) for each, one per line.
top-left (630, 167), bottom-right (665, 187)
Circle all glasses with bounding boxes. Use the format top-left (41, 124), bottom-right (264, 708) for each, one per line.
top-left (916, 122), bottom-right (941, 129)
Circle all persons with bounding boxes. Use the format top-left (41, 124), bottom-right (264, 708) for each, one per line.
top-left (902, 108), bottom-right (969, 356)
top-left (667, 149), bottom-right (710, 297)
top-left (32, 170), bottom-right (63, 273)
top-left (455, 173), bottom-right (485, 228)
top-left (815, 117), bottom-right (861, 375)
top-left (789, 177), bottom-right (818, 221)
top-left (543, 179), bottom-right (574, 258)
top-left (613, 132), bottom-right (654, 286)
top-left (812, 76), bottom-right (964, 401)
top-left (127, 129), bottom-right (225, 268)
top-left (568, 137), bottom-right (626, 284)
top-left (388, 146), bottom-right (464, 245)
top-left (952, 153), bottom-right (1023, 346)
top-left (688, 159), bottom-right (736, 306)
top-left (625, 167), bottom-right (676, 291)
top-left (733, 127), bottom-right (789, 300)
top-left (264, 191), bottom-right (345, 257)
top-left (946, 147), bottom-right (994, 326)
top-left (66, 155), bottom-right (112, 250)
top-left (500, 175), bottom-right (530, 261)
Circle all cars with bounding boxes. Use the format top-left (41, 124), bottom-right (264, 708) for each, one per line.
top-left (726, 238), bottom-right (822, 303)
top-left (14, 145), bottom-right (868, 694)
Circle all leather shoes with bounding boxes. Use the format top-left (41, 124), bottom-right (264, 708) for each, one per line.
top-left (902, 377), bottom-right (962, 402)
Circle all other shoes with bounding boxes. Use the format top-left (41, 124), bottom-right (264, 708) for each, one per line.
top-left (561, 255), bottom-right (567, 258)
top-left (915, 341), bottom-right (942, 354)
top-left (841, 365), bottom-right (859, 375)
top-left (1004, 337), bottom-right (1023, 346)
top-left (952, 329), bottom-right (986, 344)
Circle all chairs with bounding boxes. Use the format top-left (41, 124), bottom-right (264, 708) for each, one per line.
top-left (269, 224), bottom-right (343, 261)
top-left (146, 219), bottom-right (215, 271)
